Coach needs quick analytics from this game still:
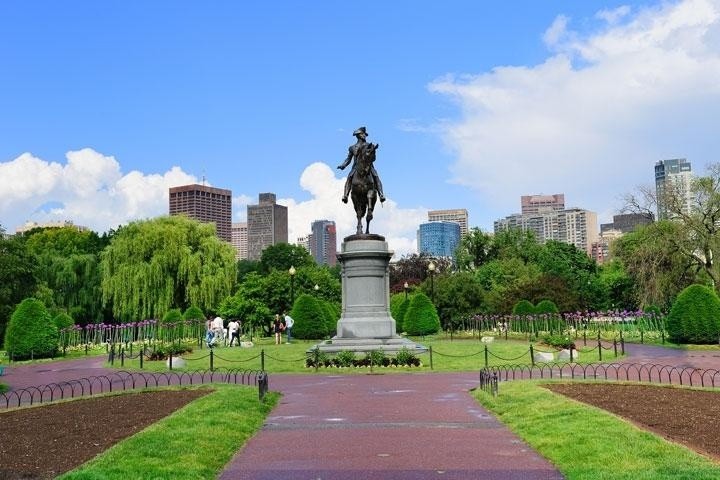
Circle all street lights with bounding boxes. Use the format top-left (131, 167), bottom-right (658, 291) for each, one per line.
top-left (428, 261), bottom-right (436, 303)
top-left (313, 283), bottom-right (320, 299)
top-left (403, 281), bottom-right (409, 299)
top-left (288, 265), bottom-right (296, 307)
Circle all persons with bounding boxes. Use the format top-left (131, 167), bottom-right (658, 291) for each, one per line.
top-left (335, 126), bottom-right (387, 203)
top-left (283, 311), bottom-right (295, 345)
top-left (204, 314), bottom-right (241, 349)
top-left (274, 313), bottom-right (284, 346)
